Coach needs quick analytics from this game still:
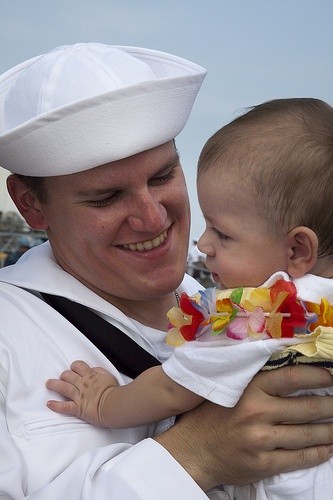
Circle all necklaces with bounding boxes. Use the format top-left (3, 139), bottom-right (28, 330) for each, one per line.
top-left (174, 290), bottom-right (180, 307)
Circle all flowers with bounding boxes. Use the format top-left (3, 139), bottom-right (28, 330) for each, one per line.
top-left (166, 280), bottom-right (333, 345)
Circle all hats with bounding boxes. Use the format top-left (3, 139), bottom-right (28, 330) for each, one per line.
top-left (0, 43), bottom-right (206, 177)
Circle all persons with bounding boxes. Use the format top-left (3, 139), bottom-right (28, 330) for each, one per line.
top-left (0, 44), bottom-right (333, 500)
top-left (45, 98), bottom-right (333, 500)
